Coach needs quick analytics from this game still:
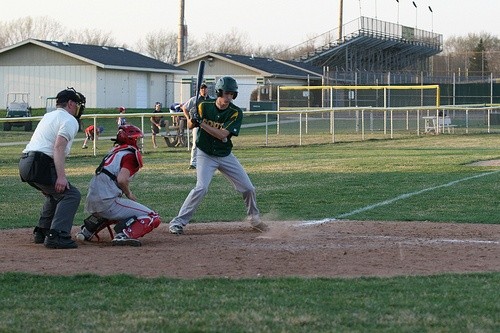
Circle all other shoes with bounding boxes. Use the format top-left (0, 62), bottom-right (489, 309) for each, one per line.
top-left (175, 141), bottom-right (181, 147)
top-left (188, 165), bottom-right (196, 170)
top-left (81, 145), bottom-right (86, 149)
top-left (182, 142), bottom-right (185, 146)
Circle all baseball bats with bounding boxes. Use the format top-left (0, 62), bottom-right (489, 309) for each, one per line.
top-left (195, 60), bottom-right (205, 115)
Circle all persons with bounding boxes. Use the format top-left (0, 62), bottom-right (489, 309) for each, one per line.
top-left (117, 107), bottom-right (126, 127)
top-left (182, 83), bottom-right (215, 169)
top-left (168, 77), bottom-right (271, 235)
top-left (82, 125), bottom-right (103, 150)
top-left (75, 125), bottom-right (162, 247)
top-left (18, 87), bottom-right (86, 249)
top-left (150, 102), bottom-right (169, 148)
top-left (169, 103), bottom-right (183, 127)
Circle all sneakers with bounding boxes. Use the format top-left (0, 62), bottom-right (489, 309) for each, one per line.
top-left (44, 230), bottom-right (79, 249)
top-left (112, 232), bottom-right (141, 246)
top-left (76, 227), bottom-right (95, 241)
top-left (34, 230), bottom-right (47, 243)
top-left (253, 221), bottom-right (269, 232)
top-left (169, 226), bottom-right (183, 234)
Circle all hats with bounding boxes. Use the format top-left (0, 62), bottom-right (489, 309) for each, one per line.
top-left (120, 107), bottom-right (125, 111)
top-left (200, 83), bottom-right (207, 89)
top-left (57, 90), bottom-right (82, 104)
top-left (154, 102), bottom-right (161, 106)
top-left (98, 127), bottom-right (103, 134)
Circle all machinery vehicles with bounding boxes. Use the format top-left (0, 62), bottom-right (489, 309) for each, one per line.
top-left (4, 90), bottom-right (32, 132)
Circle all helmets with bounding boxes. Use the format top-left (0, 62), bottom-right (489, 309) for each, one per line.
top-left (215, 77), bottom-right (238, 94)
top-left (117, 124), bottom-right (144, 150)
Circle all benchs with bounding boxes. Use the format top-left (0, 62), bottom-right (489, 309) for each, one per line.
top-left (439, 125), bottom-right (463, 134)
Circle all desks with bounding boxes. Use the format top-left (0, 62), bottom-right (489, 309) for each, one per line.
top-left (423, 115), bottom-right (454, 135)
